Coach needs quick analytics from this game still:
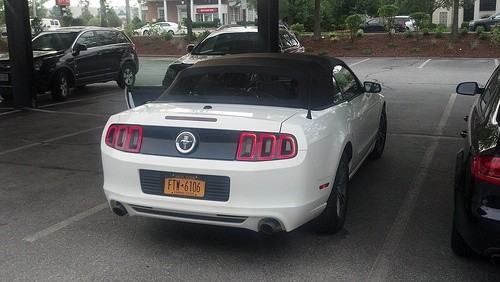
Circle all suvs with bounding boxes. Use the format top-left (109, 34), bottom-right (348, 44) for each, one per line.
top-left (161, 22), bottom-right (305, 93)
top-left (0, 25), bottom-right (140, 104)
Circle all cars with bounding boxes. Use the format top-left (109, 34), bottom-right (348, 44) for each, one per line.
top-left (450, 64), bottom-right (500, 264)
top-left (99, 51), bottom-right (388, 237)
top-left (468, 11), bottom-right (500, 32)
top-left (357, 16), bottom-right (406, 34)
top-left (388, 15), bottom-right (420, 32)
top-left (132, 21), bottom-right (187, 36)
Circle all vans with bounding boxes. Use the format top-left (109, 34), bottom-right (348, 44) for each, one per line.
top-left (30, 17), bottom-right (61, 34)
